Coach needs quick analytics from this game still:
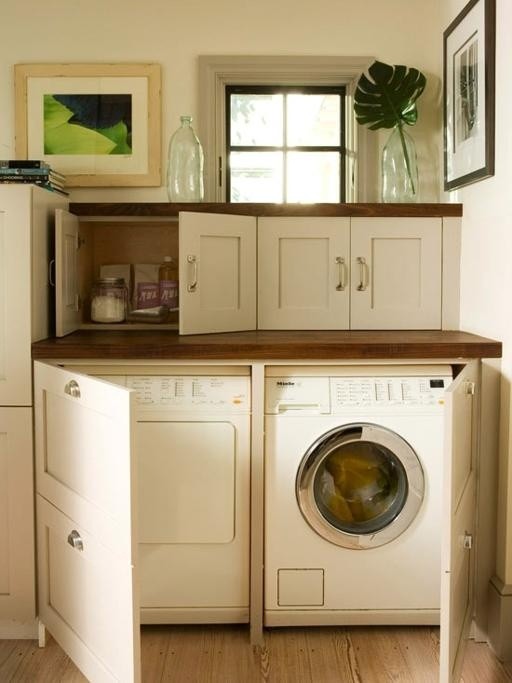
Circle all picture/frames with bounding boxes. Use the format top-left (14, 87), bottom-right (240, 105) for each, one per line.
top-left (443, 0), bottom-right (495, 191)
top-left (14, 63), bottom-right (163, 189)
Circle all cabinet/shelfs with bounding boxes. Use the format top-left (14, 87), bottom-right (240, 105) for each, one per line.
top-left (49, 211), bottom-right (257, 337)
top-left (257, 216), bottom-right (442, 332)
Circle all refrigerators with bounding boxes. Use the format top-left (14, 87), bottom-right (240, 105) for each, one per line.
top-left (2, 184), bottom-right (66, 644)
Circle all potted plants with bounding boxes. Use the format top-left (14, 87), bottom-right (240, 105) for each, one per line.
top-left (354, 60), bottom-right (426, 203)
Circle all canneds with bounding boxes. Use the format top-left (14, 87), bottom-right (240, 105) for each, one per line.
top-left (91, 278), bottom-right (127, 322)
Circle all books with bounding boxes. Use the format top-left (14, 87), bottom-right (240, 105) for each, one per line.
top-left (1, 158), bottom-right (74, 196)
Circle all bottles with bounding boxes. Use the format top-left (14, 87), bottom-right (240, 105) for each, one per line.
top-left (160, 257), bottom-right (179, 312)
top-left (168, 114), bottom-right (204, 203)
top-left (85, 278), bottom-right (129, 324)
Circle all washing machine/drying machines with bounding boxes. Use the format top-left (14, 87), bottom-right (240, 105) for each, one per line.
top-left (269, 370), bottom-right (456, 608)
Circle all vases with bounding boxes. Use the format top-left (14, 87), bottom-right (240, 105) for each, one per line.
top-left (166, 117), bottom-right (207, 203)
